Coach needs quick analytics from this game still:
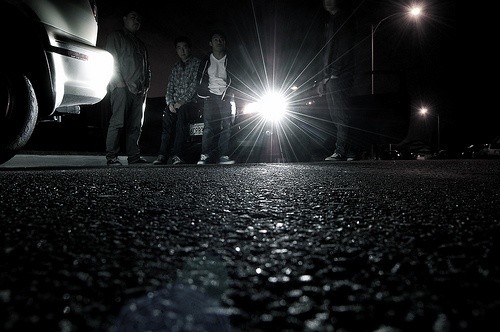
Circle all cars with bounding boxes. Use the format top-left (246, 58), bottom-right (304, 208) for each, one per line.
top-left (187, 73), bottom-right (297, 164)
top-left (0, 0), bottom-right (116, 165)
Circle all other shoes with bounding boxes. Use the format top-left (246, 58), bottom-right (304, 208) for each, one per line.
top-left (197, 153), bottom-right (216, 165)
top-left (106, 158), bottom-right (122, 165)
top-left (128, 158), bottom-right (157, 167)
top-left (218, 155), bottom-right (235, 164)
top-left (172, 157), bottom-right (183, 165)
top-left (325, 152), bottom-right (345, 161)
top-left (346, 157), bottom-right (356, 161)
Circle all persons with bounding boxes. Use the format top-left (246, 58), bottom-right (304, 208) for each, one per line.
top-left (153, 37), bottom-right (202, 166)
top-left (104, 8), bottom-right (151, 166)
top-left (315, 0), bottom-right (367, 162)
top-left (196, 31), bottom-right (237, 165)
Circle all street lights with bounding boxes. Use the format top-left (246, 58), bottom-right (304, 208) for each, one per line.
top-left (372, 6), bottom-right (421, 95)
